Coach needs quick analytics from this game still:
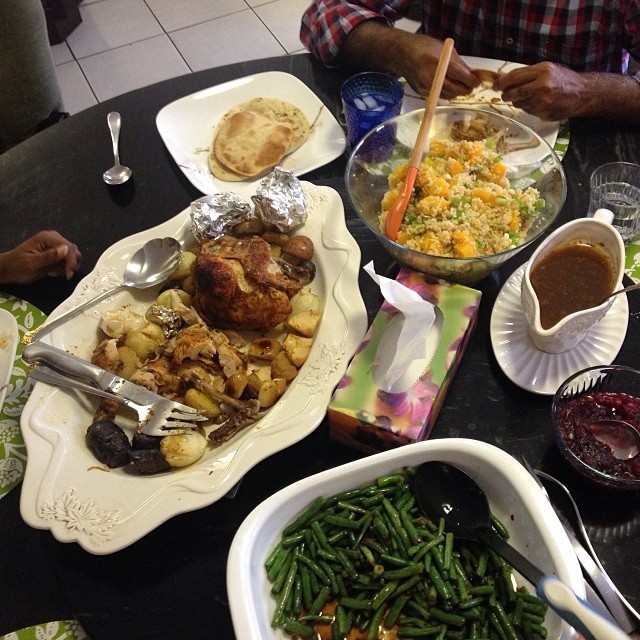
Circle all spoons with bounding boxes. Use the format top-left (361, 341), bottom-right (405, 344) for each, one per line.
top-left (102, 111), bottom-right (132, 186)
top-left (30, 237), bottom-right (182, 343)
top-left (587, 420), bottom-right (639, 461)
top-left (414, 460), bottom-right (632, 639)
top-left (385, 37), bottom-right (455, 241)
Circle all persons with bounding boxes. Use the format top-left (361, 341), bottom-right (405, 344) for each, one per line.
top-left (298, 0), bottom-right (640, 122)
top-left (0, 227), bottom-right (84, 281)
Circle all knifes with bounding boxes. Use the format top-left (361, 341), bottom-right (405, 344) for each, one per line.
top-left (522, 454), bottom-right (639, 635)
top-left (22, 341), bottom-right (208, 421)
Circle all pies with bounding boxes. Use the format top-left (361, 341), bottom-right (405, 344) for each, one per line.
top-left (208, 96), bottom-right (311, 182)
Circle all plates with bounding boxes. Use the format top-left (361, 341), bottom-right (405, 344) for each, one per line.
top-left (19, 176), bottom-right (370, 557)
top-left (155, 70), bottom-right (347, 196)
top-left (396, 55), bottom-right (561, 166)
top-left (489, 260), bottom-right (630, 397)
top-left (0, 307), bottom-right (19, 413)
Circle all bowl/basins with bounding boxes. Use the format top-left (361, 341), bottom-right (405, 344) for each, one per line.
top-left (343, 106), bottom-right (568, 286)
top-left (226, 437), bottom-right (588, 640)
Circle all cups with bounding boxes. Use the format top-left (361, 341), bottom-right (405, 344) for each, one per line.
top-left (550, 364), bottom-right (640, 497)
top-left (587, 161), bottom-right (640, 247)
top-left (521, 207), bottom-right (626, 355)
top-left (339, 70), bottom-right (404, 163)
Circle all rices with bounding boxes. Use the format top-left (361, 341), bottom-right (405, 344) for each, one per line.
top-left (378, 140), bottom-right (547, 280)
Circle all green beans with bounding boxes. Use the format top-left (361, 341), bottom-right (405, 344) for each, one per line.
top-left (263, 468), bottom-right (551, 640)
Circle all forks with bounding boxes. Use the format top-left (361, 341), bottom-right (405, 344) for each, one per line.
top-left (531, 466), bottom-right (639, 623)
top-left (26, 363), bottom-right (208, 435)
